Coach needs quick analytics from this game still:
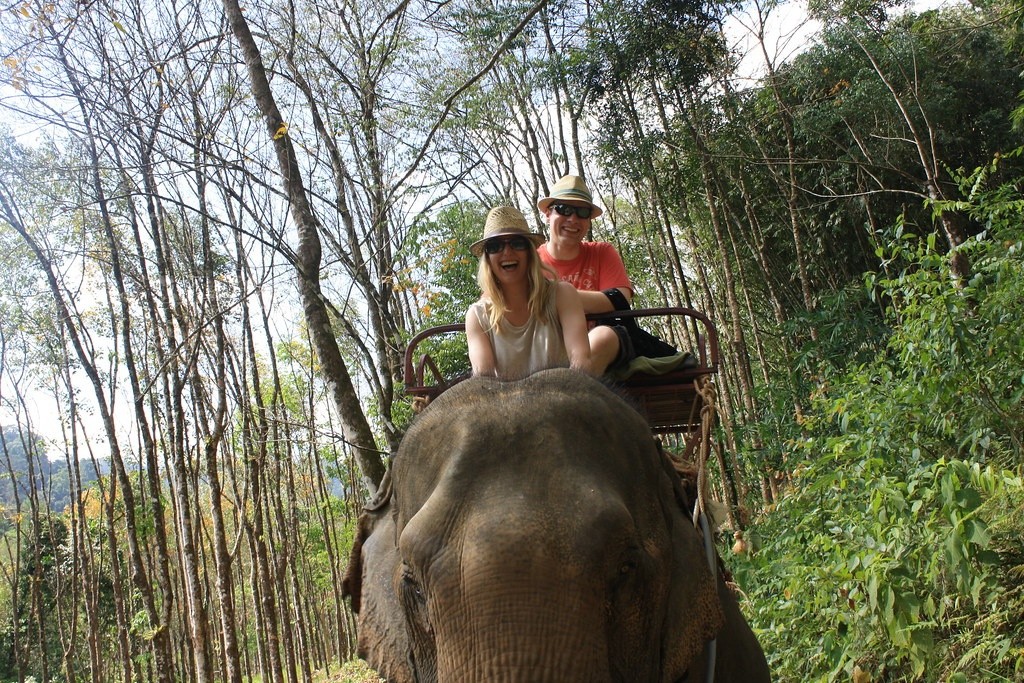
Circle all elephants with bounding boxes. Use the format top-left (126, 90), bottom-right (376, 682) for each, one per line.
top-left (339, 364), bottom-right (773, 683)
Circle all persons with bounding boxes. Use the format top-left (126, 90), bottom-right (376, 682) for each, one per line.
top-left (465, 206), bottom-right (590, 377)
top-left (535, 175), bottom-right (635, 379)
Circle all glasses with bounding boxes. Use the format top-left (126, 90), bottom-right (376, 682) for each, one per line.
top-left (484, 236), bottom-right (530, 255)
top-left (548, 203), bottom-right (592, 219)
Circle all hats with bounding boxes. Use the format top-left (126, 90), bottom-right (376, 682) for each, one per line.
top-left (536, 174), bottom-right (603, 220)
top-left (468, 206), bottom-right (546, 258)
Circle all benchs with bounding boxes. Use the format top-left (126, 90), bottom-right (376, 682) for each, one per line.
top-left (402, 308), bottom-right (719, 463)
